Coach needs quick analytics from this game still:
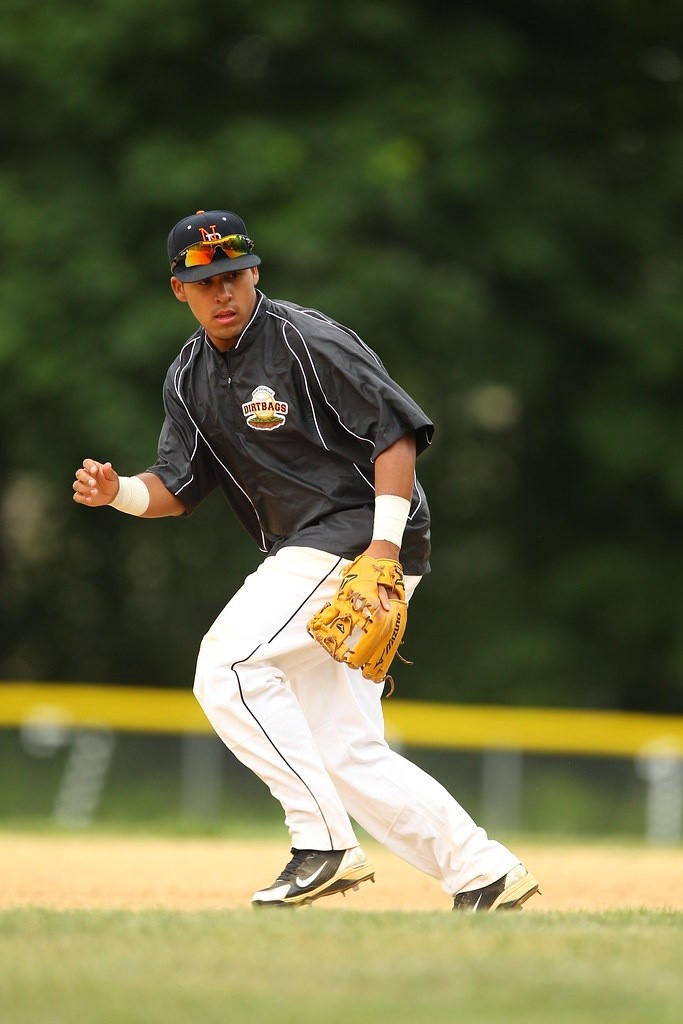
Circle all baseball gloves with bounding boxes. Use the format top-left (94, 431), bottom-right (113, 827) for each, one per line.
top-left (307, 556), bottom-right (409, 684)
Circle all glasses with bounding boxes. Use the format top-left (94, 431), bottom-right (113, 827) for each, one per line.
top-left (170, 234), bottom-right (254, 274)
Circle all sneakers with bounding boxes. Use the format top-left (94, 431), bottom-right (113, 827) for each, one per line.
top-left (249, 845), bottom-right (376, 908)
top-left (453, 863), bottom-right (539, 913)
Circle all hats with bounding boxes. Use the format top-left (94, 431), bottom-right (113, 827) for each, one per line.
top-left (167, 210), bottom-right (262, 284)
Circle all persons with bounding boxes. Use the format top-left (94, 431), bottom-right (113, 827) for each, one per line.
top-left (71, 209), bottom-right (540, 914)
